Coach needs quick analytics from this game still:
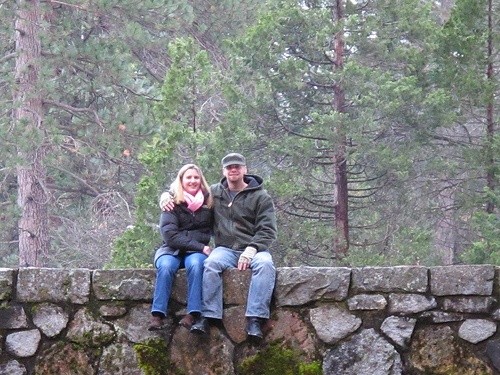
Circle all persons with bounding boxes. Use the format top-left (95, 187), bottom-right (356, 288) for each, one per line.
top-left (148, 164), bottom-right (213, 331)
top-left (160, 153), bottom-right (276, 339)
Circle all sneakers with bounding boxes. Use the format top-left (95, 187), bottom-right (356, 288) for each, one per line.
top-left (244, 318), bottom-right (266, 339)
top-left (148, 313), bottom-right (168, 331)
top-left (177, 313), bottom-right (194, 329)
top-left (191, 316), bottom-right (208, 334)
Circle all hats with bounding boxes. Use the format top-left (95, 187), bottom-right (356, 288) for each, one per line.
top-left (221, 153), bottom-right (246, 166)
